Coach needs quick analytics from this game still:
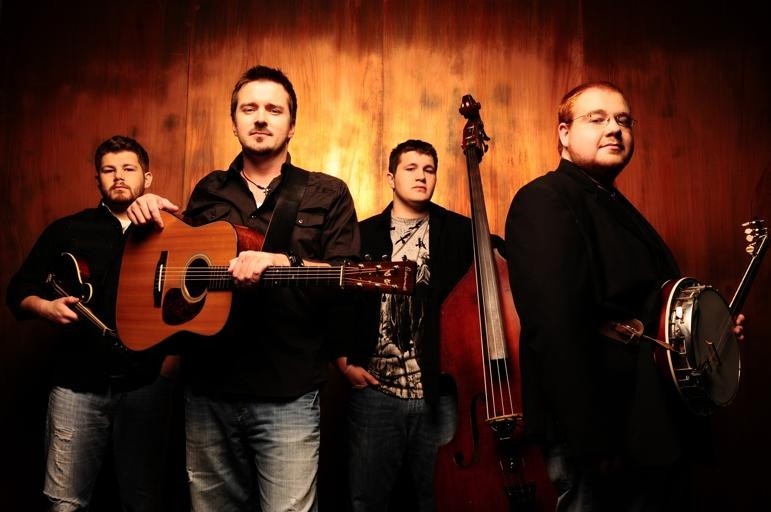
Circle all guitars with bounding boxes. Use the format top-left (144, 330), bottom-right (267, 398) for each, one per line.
top-left (115, 212), bottom-right (418, 351)
top-left (44, 253), bottom-right (118, 349)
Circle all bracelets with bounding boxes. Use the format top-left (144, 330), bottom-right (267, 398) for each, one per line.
top-left (343, 364), bottom-right (353, 375)
top-left (284, 248), bottom-right (304, 267)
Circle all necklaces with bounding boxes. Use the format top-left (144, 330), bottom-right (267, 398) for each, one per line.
top-left (241, 169), bottom-right (270, 197)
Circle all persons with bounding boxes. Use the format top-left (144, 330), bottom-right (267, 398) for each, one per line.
top-left (327, 140), bottom-right (477, 512)
top-left (5, 137), bottom-right (172, 511)
top-left (123, 65), bottom-right (363, 512)
top-left (504, 80), bottom-right (747, 512)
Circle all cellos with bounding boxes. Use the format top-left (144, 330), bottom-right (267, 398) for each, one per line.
top-left (434, 94), bottom-right (559, 509)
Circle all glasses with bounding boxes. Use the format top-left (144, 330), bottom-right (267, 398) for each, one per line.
top-left (567, 113), bottom-right (639, 129)
